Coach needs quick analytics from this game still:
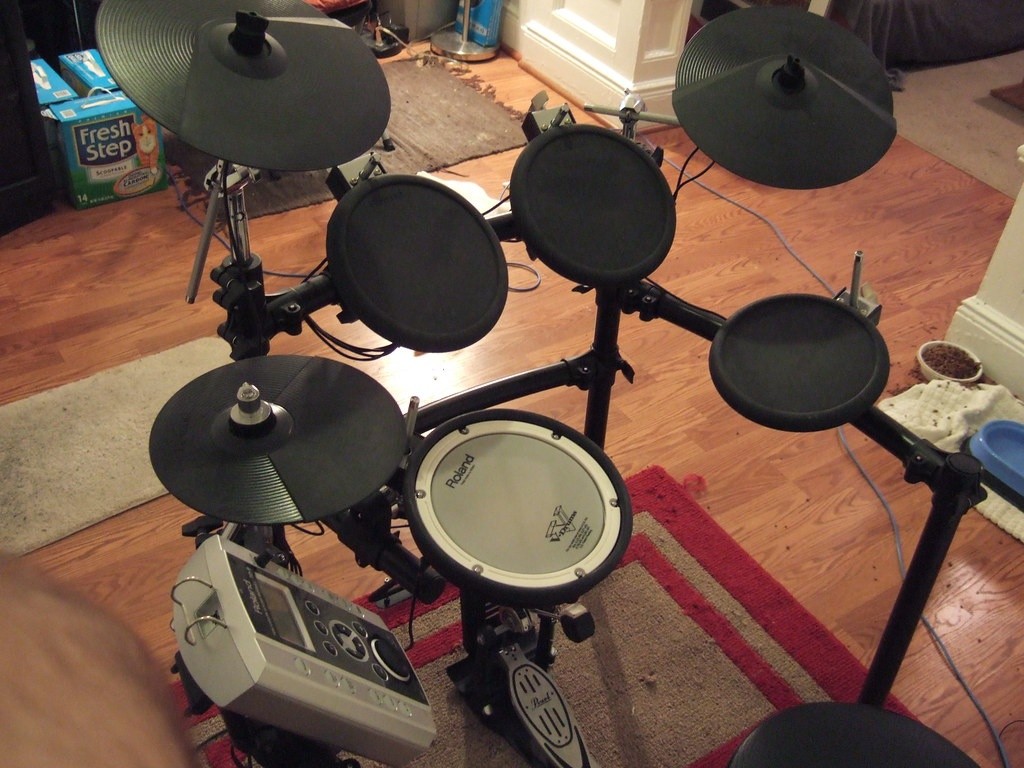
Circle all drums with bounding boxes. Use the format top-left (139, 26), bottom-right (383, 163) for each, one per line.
top-left (404, 408), bottom-right (633, 610)
top-left (710, 293), bottom-right (890, 432)
top-left (510, 125), bottom-right (676, 287)
top-left (325, 174), bottom-right (508, 353)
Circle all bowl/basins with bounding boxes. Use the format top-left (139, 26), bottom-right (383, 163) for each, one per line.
top-left (916, 341), bottom-right (984, 384)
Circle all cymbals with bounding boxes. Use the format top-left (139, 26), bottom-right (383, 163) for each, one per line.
top-left (671, 5), bottom-right (897, 190)
top-left (95, 1), bottom-right (391, 173)
top-left (149, 354), bottom-right (405, 525)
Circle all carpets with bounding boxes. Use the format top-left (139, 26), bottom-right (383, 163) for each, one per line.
top-left (160, 58), bottom-right (535, 227)
top-left (161, 462), bottom-right (919, 768)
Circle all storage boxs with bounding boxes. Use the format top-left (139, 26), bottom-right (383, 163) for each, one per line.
top-left (26, 46), bottom-right (169, 208)
